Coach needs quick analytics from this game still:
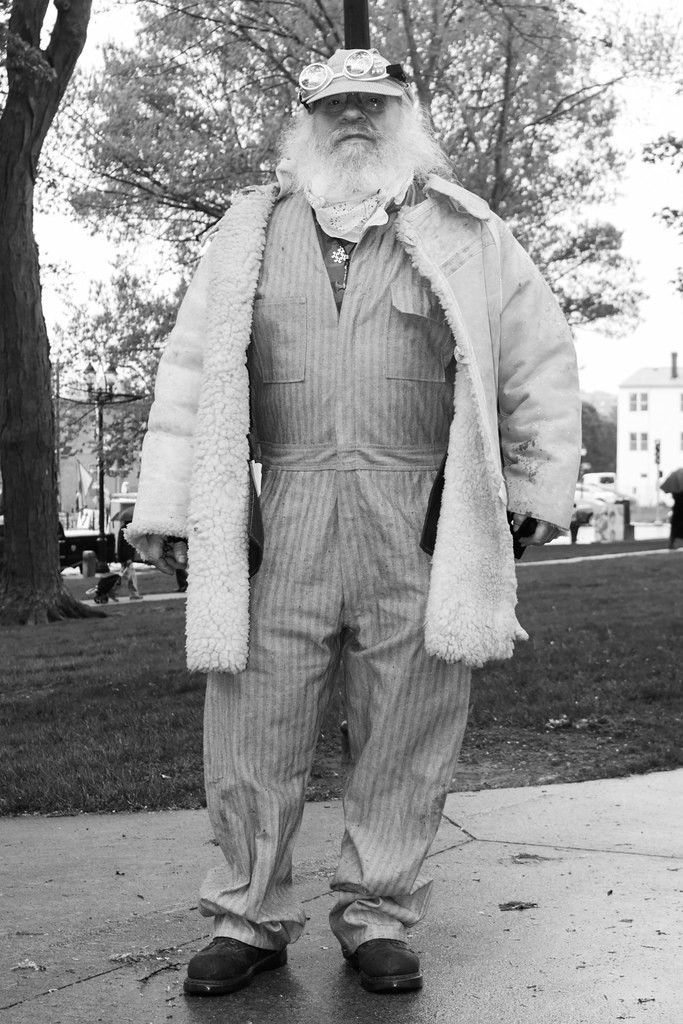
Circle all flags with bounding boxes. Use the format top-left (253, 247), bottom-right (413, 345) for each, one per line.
top-left (76, 461), bottom-right (94, 510)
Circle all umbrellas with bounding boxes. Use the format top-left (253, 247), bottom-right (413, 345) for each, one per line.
top-left (659, 467), bottom-right (683, 495)
top-left (111, 505), bottom-right (135, 522)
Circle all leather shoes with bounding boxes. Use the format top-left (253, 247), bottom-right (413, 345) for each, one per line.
top-left (183, 937), bottom-right (287, 994)
top-left (341, 936), bottom-right (423, 990)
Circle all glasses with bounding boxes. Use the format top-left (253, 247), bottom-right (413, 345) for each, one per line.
top-left (314, 91), bottom-right (389, 115)
top-left (297, 51), bottom-right (407, 104)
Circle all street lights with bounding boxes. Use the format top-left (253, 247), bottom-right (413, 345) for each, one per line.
top-left (84, 361), bottom-right (118, 576)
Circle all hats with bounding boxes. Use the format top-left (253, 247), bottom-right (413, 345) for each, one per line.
top-left (305, 49), bottom-right (416, 108)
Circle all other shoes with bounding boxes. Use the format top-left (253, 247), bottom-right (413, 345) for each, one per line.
top-left (129, 594), bottom-right (144, 600)
top-left (107, 591), bottom-right (120, 602)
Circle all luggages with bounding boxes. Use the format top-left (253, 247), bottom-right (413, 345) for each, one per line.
top-left (95, 562), bottom-right (131, 603)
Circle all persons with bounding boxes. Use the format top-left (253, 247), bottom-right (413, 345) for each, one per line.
top-left (58, 521), bottom-right (68, 571)
top-left (120, 45), bottom-right (583, 996)
top-left (666, 488), bottom-right (683, 550)
top-left (569, 503), bottom-right (581, 543)
top-left (109, 520), bottom-right (143, 602)
top-left (176, 568), bottom-right (189, 593)
